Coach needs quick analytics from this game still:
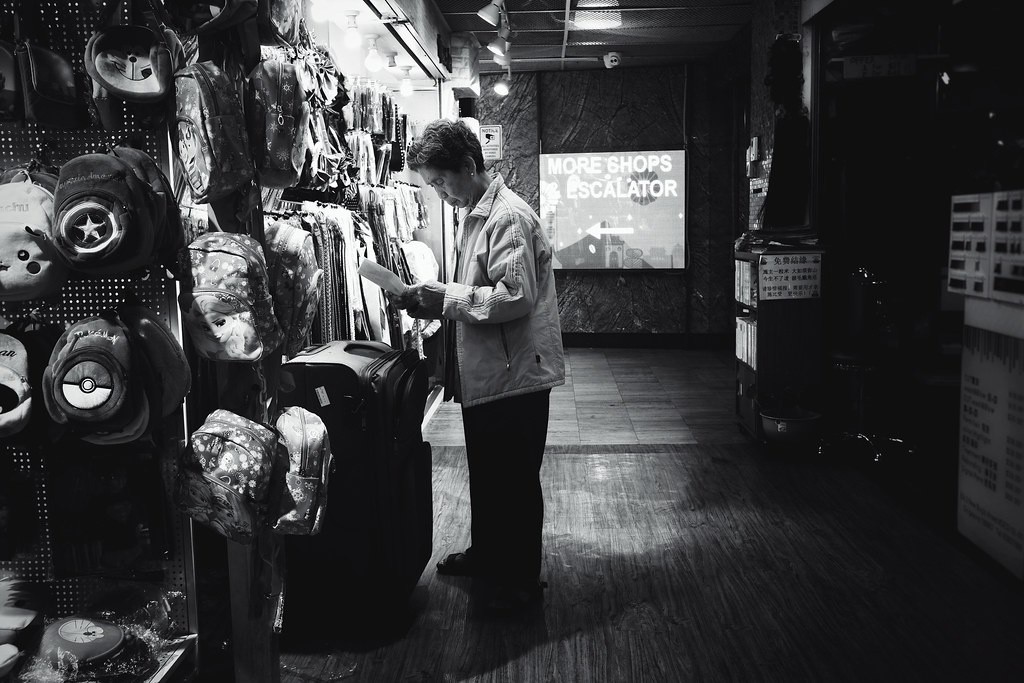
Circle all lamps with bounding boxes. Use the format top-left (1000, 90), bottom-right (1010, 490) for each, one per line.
top-left (477, 0), bottom-right (512, 96)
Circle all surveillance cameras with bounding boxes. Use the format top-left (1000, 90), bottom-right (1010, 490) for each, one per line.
top-left (609, 51), bottom-right (622, 66)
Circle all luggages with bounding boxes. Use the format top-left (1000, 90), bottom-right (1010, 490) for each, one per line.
top-left (277, 334), bottom-right (435, 654)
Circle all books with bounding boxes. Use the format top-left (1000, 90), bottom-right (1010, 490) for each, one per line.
top-left (734, 260), bottom-right (758, 308)
top-left (735, 316), bottom-right (758, 371)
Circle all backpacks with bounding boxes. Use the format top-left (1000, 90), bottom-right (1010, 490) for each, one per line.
top-left (0, 1), bottom-right (341, 683)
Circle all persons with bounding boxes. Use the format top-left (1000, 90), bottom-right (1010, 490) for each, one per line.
top-left (384, 118), bottom-right (567, 622)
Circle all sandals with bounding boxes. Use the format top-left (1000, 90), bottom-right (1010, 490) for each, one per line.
top-left (438, 549), bottom-right (478, 575)
top-left (486, 583), bottom-right (537, 617)
